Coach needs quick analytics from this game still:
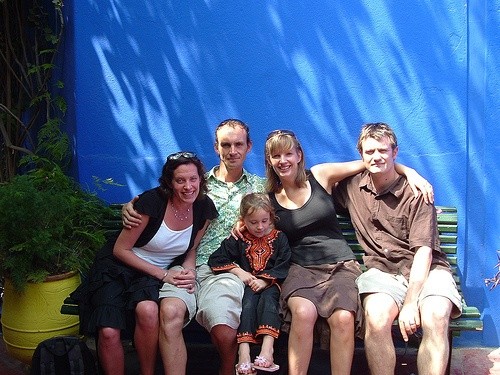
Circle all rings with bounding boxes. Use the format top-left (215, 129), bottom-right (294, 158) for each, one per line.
top-left (410, 321), bottom-right (415, 325)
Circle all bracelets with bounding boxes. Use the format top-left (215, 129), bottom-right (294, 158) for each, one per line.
top-left (189, 268), bottom-right (197, 274)
top-left (161, 270), bottom-right (169, 280)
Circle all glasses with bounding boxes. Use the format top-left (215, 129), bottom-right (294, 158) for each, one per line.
top-left (167, 152), bottom-right (197, 161)
top-left (266, 129), bottom-right (295, 142)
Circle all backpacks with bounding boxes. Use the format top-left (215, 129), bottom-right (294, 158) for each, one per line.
top-left (31, 337), bottom-right (96, 375)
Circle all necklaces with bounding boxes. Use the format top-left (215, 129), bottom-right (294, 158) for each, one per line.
top-left (171, 201), bottom-right (190, 220)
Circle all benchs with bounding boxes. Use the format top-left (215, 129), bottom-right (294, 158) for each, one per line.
top-left (62, 207), bottom-right (483, 375)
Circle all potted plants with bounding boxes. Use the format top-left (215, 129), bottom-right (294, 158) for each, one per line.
top-left (0, 34), bottom-right (121, 364)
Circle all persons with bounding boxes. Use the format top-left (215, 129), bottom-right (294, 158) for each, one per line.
top-left (70, 151), bottom-right (220, 375)
top-left (227, 130), bottom-right (434, 375)
top-left (208, 192), bottom-right (292, 375)
top-left (334, 122), bottom-right (462, 375)
top-left (121, 119), bottom-right (268, 375)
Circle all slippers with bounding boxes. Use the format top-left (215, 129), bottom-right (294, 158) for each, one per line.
top-left (252, 357), bottom-right (280, 372)
top-left (236, 362), bottom-right (257, 375)
